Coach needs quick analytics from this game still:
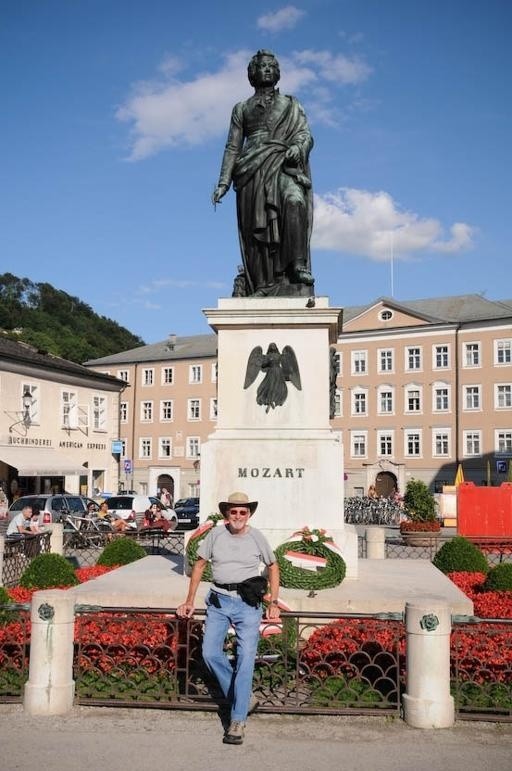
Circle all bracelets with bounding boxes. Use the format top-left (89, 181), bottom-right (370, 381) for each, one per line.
top-left (270, 600), bottom-right (278, 605)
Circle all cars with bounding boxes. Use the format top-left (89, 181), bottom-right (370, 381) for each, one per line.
top-left (103, 494), bottom-right (178, 530)
top-left (174, 498), bottom-right (201, 527)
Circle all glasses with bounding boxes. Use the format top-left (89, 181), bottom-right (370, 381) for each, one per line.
top-left (230, 509), bottom-right (248, 516)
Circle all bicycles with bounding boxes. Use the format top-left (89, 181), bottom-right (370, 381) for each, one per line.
top-left (345, 496), bottom-right (399, 524)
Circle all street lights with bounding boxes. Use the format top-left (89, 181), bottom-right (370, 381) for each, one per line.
top-left (455, 325), bottom-right (464, 468)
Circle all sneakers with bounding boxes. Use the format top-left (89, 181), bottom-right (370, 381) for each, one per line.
top-left (248, 690), bottom-right (260, 714)
top-left (224, 720), bottom-right (246, 745)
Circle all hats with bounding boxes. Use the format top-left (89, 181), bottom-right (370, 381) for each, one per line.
top-left (219, 492), bottom-right (258, 516)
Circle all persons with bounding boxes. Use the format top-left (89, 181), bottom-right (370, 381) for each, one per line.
top-left (207, 48), bottom-right (315, 298)
top-left (1, 487), bottom-right (173, 560)
top-left (256, 342), bottom-right (290, 415)
top-left (387, 486), bottom-right (402, 503)
top-left (367, 485), bottom-right (379, 503)
top-left (174, 492), bottom-right (281, 747)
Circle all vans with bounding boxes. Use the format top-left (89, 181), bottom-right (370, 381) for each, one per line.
top-left (8, 492), bottom-right (123, 526)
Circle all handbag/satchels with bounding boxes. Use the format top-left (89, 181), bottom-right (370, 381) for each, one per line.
top-left (240, 576), bottom-right (268, 606)
